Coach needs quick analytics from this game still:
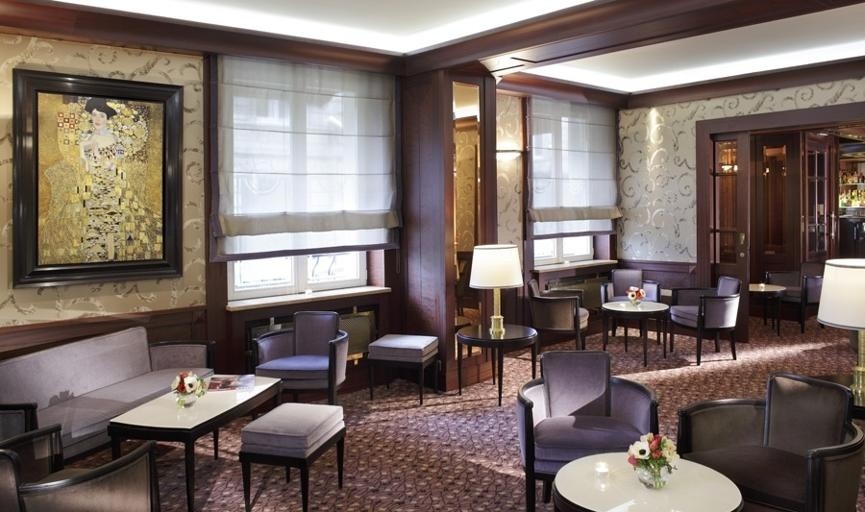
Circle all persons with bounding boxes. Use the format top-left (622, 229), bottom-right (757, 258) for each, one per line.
top-left (81, 98), bottom-right (162, 261)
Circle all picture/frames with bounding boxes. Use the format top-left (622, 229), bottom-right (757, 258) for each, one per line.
top-left (13, 68), bottom-right (184, 289)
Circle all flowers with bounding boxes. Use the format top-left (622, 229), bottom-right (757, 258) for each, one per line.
top-left (168, 369), bottom-right (207, 408)
top-left (625, 286), bottom-right (646, 300)
top-left (627, 432), bottom-right (680, 486)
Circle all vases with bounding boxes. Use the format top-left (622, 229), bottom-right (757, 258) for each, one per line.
top-left (632, 299), bottom-right (641, 307)
top-left (181, 394), bottom-right (197, 408)
top-left (638, 470), bottom-right (669, 490)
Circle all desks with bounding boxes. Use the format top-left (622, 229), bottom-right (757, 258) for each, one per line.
top-left (457, 323), bottom-right (538, 407)
top-left (810, 374), bottom-right (865, 420)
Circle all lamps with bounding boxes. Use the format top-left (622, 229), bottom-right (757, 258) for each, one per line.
top-left (817, 258), bottom-right (865, 408)
top-left (469, 244), bottom-right (524, 339)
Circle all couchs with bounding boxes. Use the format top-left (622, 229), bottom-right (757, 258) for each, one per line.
top-left (251, 310), bottom-right (350, 420)
top-left (518, 351), bottom-right (659, 511)
top-left (0, 324), bottom-right (213, 461)
top-left (758, 261), bottom-right (824, 333)
top-left (676, 372), bottom-right (865, 512)
top-left (600, 268), bottom-right (661, 344)
top-left (1, 423), bottom-right (161, 512)
top-left (670, 276), bottom-right (743, 365)
top-left (528, 279), bottom-right (590, 354)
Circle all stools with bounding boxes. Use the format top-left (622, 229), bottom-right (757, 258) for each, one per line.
top-left (239, 402), bottom-right (347, 512)
top-left (367, 333), bottom-right (438, 405)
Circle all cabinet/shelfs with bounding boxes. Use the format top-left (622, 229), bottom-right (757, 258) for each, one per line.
top-left (840, 159), bottom-right (865, 208)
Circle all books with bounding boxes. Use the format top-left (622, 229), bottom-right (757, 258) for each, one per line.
top-left (207, 374), bottom-right (255, 391)
